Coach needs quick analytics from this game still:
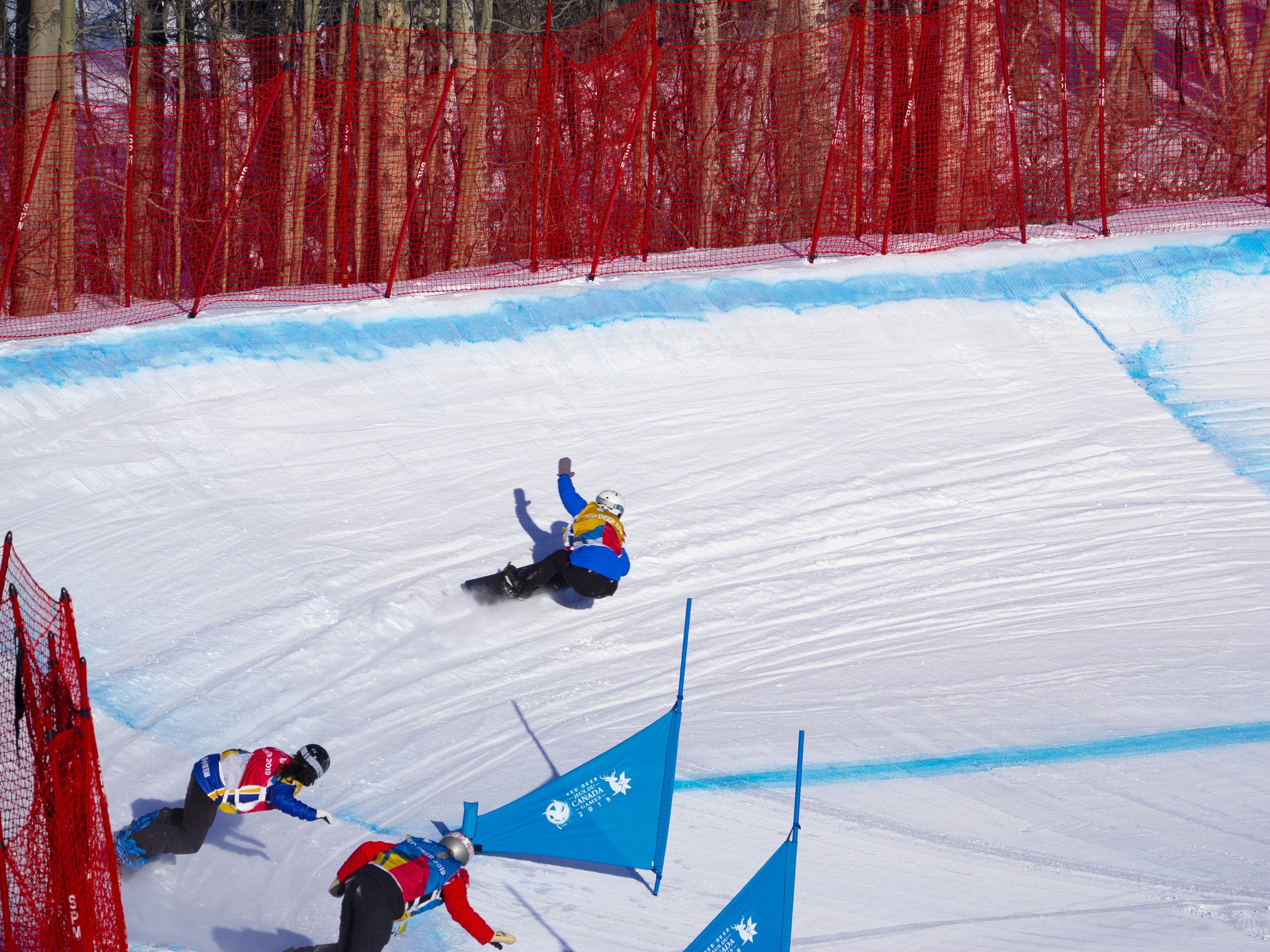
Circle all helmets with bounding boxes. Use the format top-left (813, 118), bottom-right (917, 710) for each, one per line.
top-left (595, 489), bottom-right (625, 519)
top-left (296, 744), bottom-right (330, 787)
top-left (439, 831), bottom-right (474, 867)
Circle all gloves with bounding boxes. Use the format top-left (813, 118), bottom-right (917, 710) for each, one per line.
top-left (489, 931), bottom-right (516, 945)
top-left (316, 808), bottom-right (334, 825)
top-left (557, 457), bottom-right (574, 478)
top-left (329, 877), bottom-right (345, 898)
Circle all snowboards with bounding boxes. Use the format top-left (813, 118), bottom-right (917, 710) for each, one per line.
top-left (466, 560), bottom-right (571, 606)
top-left (114, 807), bottom-right (171, 866)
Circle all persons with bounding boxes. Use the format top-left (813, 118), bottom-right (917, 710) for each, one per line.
top-left (502, 457), bottom-right (631, 600)
top-left (115, 743), bottom-right (335, 870)
top-left (281, 831), bottom-right (517, 952)
